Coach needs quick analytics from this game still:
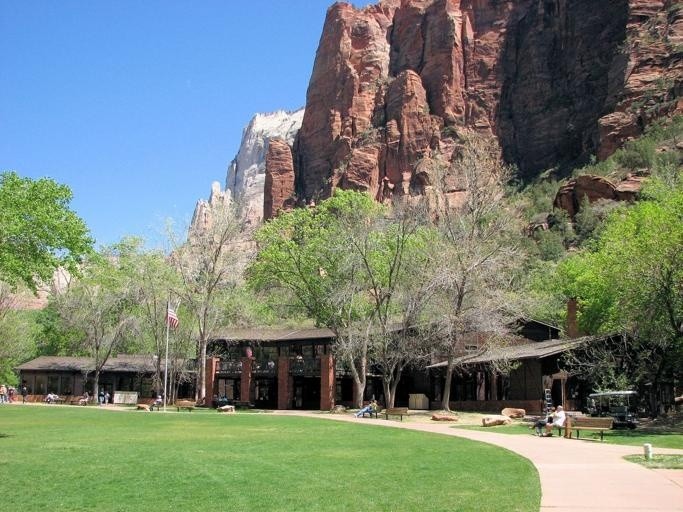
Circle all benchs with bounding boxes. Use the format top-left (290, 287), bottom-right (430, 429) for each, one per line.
top-left (22, 394), bottom-right (88, 405)
top-left (556, 415), bottom-right (614, 439)
top-left (362, 406), bottom-right (409, 422)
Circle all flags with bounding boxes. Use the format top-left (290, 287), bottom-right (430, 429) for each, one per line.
top-left (164, 290), bottom-right (181, 331)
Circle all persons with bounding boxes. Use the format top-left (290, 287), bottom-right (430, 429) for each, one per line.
top-left (0, 385), bottom-right (18, 404)
top-left (98, 387), bottom-right (105, 406)
top-left (44, 391), bottom-right (58, 403)
top-left (147, 395), bottom-right (162, 410)
top-left (77, 390), bottom-right (89, 406)
top-left (527, 406), bottom-right (555, 437)
top-left (20, 386), bottom-right (28, 405)
top-left (353, 398), bottom-right (377, 418)
top-left (541, 404), bottom-right (566, 437)
top-left (103, 390), bottom-right (110, 404)
top-left (368, 399), bottom-right (377, 416)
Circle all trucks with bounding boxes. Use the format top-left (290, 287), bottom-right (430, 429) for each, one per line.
top-left (587, 391), bottom-right (639, 429)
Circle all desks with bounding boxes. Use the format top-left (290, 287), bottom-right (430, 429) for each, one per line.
top-left (175, 400), bottom-right (197, 412)
